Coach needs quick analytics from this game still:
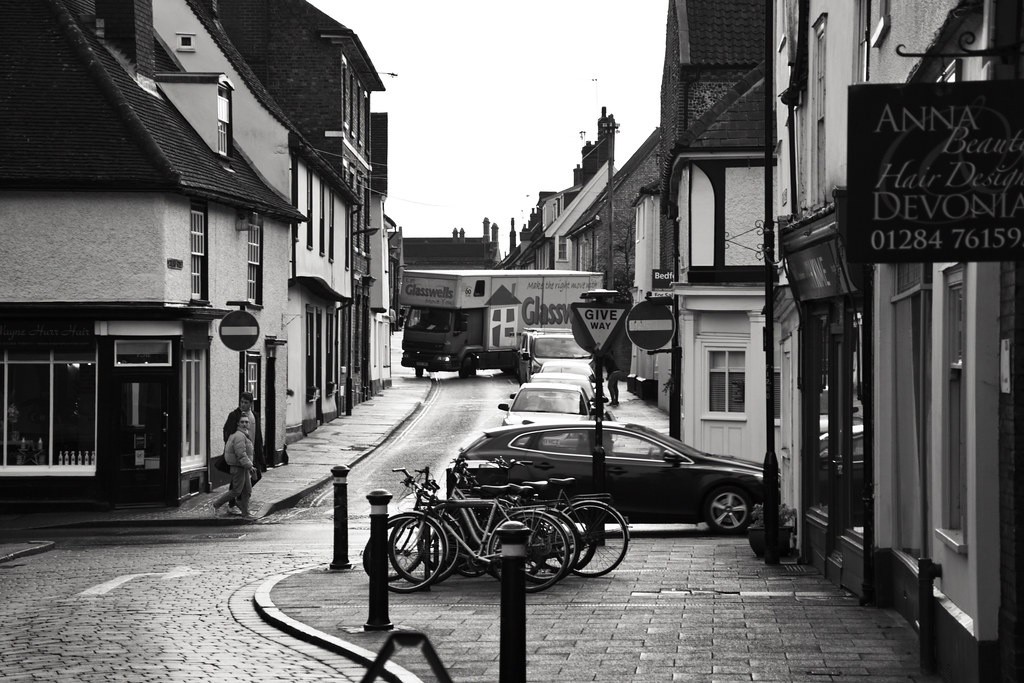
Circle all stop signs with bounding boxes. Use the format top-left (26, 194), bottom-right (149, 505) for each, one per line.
top-left (624, 301), bottom-right (675, 351)
top-left (219, 310), bottom-right (260, 352)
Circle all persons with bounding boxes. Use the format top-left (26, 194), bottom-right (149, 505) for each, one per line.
top-left (222, 392), bottom-right (267, 515)
top-left (390, 306), bottom-right (396, 336)
top-left (603, 352), bottom-right (622, 406)
top-left (209, 413), bottom-right (258, 519)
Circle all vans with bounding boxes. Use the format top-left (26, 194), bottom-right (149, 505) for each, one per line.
top-left (512, 327), bottom-right (594, 387)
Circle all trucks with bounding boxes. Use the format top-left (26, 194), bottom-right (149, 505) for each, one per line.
top-left (397, 264), bottom-right (607, 380)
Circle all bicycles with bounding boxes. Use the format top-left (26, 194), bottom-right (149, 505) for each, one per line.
top-left (363, 453), bottom-right (630, 594)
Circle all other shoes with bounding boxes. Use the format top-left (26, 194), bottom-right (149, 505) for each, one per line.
top-left (209, 503), bottom-right (217, 518)
top-left (227, 504), bottom-right (242, 514)
top-left (244, 514), bottom-right (257, 520)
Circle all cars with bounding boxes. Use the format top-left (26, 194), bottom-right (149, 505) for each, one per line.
top-left (509, 359), bottom-right (609, 413)
top-left (450, 420), bottom-right (767, 536)
top-left (496, 382), bottom-right (598, 450)
